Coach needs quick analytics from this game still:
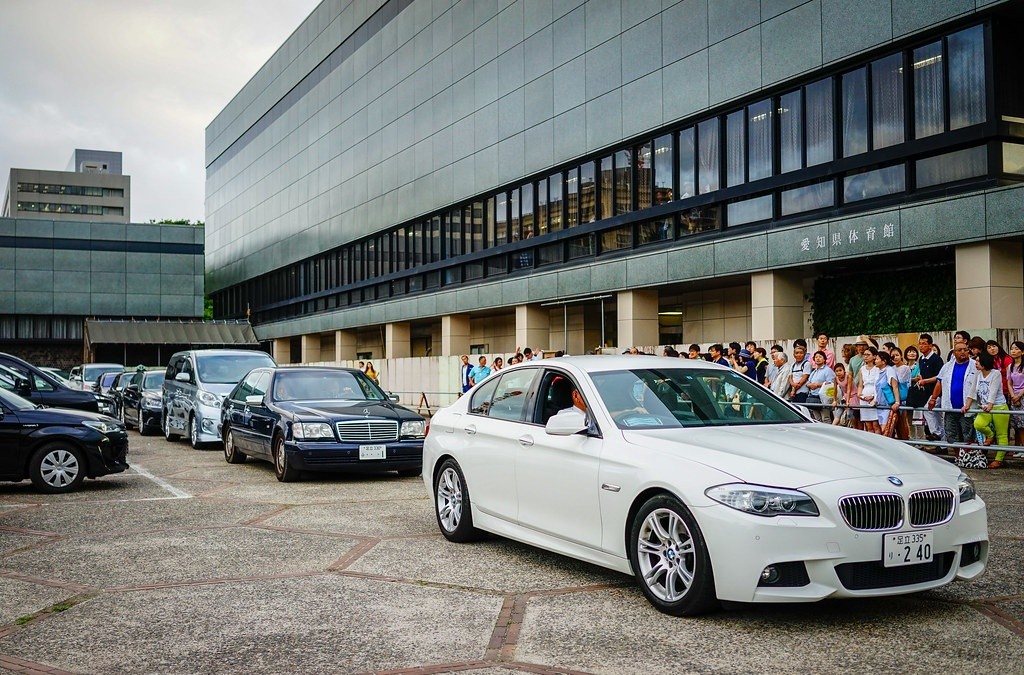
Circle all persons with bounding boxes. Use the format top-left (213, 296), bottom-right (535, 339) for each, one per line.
top-left (555, 351), bottom-right (570, 357)
top-left (461, 347), bottom-right (543, 398)
top-left (359, 361), bottom-right (379, 386)
top-left (557, 386), bottom-right (649, 435)
top-left (663, 330), bottom-right (1024, 468)
top-left (622, 347), bottom-right (646, 355)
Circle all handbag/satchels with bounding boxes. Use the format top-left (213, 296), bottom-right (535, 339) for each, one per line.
top-left (818, 381), bottom-right (842, 404)
top-left (958, 448), bottom-right (988, 468)
top-left (806, 397), bottom-right (821, 408)
top-left (906, 386), bottom-right (929, 408)
top-left (840, 410), bottom-right (848, 425)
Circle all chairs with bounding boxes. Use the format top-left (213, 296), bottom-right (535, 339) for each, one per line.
top-left (542, 378), bottom-right (573, 424)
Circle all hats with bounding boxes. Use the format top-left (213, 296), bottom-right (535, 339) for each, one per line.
top-left (853, 336), bottom-right (867, 345)
top-left (622, 348), bottom-right (630, 354)
top-left (739, 349), bottom-right (750, 358)
top-left (722, 348), bottom-right (730, 356)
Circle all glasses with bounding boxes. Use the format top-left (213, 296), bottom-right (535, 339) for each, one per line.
top-left (955, 337), bottom-right (963, 339)
top-left (920, 342), bottom-right (928, 346)
top-left (863, 354), bottom-right (872, 356)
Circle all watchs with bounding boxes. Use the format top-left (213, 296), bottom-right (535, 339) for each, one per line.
top-left (792, 389), bottom-right (795, 392)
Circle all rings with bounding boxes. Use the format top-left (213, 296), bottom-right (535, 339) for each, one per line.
top-left (892, 404), bottom-right (895, 408)
top-left (987, 408), bottom-right (989, 411)
top-left (812, 388), bottom-right (813, 389)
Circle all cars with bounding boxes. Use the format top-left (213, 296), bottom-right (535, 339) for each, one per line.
top-left (422, 353), bottom-right (992, 619)
top-left (0, 387), bottom-right (130, 494)
top-left (0, 352), bottom-right (167, 437)
top-left (216, 364), bottom-right (427, 483)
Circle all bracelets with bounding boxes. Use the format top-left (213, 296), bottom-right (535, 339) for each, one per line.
top-left (377, 375), bottom-right (378, 376)
top-left (861, 396), bottom-right (867, 400)
top-left (895, 402), bottom-right (900, 405)
top-left (471, 383), bottom-right (474, 386)
top-left (1020, 392), bottom-right (1023, 397)
top-left (833, 400), bottom-right (837, 403)
top-left (806, 384), bottom-right (809, 387)
top-left (930, 399), bottom-right (937, 401)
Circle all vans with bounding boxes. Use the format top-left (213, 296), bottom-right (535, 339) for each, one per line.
top-left (159, 348), bottom-right (281, 450)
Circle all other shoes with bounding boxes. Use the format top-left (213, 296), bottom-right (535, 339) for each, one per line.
top-left (988, 461), bottom-right (1003, 468)
top-left (924, 446), bottom-right (948, 453)
top-left (914, 445), bottom-right (924, 450)
top-left (926, 434), bottom-right (940, 440)
top-left (971, 441), bottom-right (978, 445)
top-left (1013, 452), bottom-right (1024, 457)
top-left (984, 436), bottom-right (996, 445)
